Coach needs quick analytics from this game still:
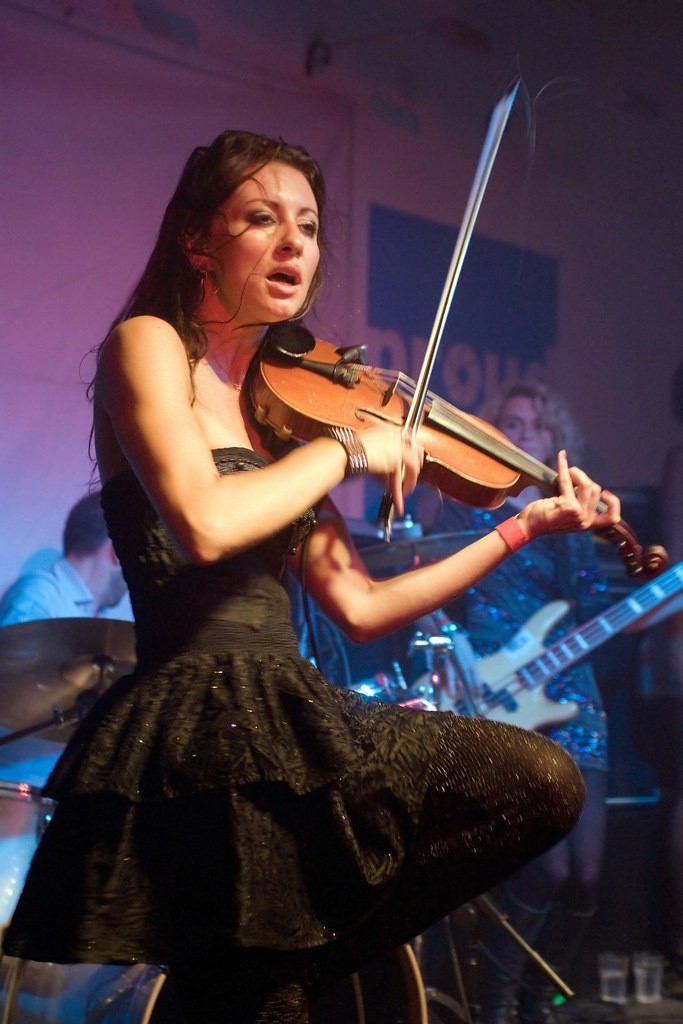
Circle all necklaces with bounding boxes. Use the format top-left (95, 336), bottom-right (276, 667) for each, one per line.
top-left (207, 346), bottom-right (245, 391)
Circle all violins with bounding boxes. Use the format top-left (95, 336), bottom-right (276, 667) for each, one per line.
top-left (239, 317), bottom-right (676, 588)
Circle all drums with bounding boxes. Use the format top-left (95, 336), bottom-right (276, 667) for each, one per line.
top-left (0, 780), bottom-right (57, 927)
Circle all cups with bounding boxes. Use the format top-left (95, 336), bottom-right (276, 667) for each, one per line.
top-left (596, 953), bottom-right (630, 1004)
top-left (632, 951), bottom-right (665, 1003)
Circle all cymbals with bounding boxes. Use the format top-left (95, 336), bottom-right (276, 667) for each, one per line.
top-left (0, 619), bottom-right (138, 738)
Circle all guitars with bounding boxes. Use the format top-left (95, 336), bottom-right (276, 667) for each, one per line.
top-left (408, 551), bottom-right (682, 731)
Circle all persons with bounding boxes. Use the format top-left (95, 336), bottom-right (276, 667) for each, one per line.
top-left (0, 130), bottom-right (683, 1024)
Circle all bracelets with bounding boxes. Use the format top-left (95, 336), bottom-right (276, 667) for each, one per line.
top-left (326, 424), bottom-right (370, 480)
top-left (491, 516), bottom-right (527, 557)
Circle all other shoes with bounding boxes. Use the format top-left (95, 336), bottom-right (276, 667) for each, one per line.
top-left (521, 982), bottom-right (621, 1024)
top-left (478, 981), bottom-right (514, 1024)
